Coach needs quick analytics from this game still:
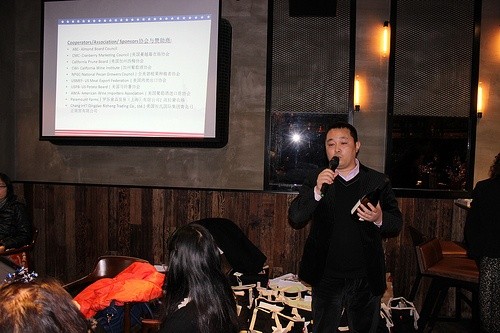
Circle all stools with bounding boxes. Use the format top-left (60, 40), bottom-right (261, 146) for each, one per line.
top-left (403, 226), bottom-right (480, 333)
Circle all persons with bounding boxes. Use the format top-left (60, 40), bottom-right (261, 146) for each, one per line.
top-left (286, 123), bottom-right (404, 333)
top-left (462, 152), bottom-right (500, 333)
top-left (189, 217), bottom-right (267, 286)
top-left (157, 224), bottom-right (241, 333)
top-left (0, 276), bottom-right (91, 333)
top-left (0, 172), bottom-right (32, 253)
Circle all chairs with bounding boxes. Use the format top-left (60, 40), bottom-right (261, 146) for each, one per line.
top-left (62, 256), bottom-right (144, 333)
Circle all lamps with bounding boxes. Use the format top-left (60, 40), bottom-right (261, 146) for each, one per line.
top-left (382, 21), bottom-right (390, 55)
top-left (477, 81), bottom-right (486, 119)
top-left (355, 75), bottom-right (361, 113)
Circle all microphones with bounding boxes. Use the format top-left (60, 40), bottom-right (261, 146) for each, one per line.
top-left (321, 156), bottom-right (339, 197)
